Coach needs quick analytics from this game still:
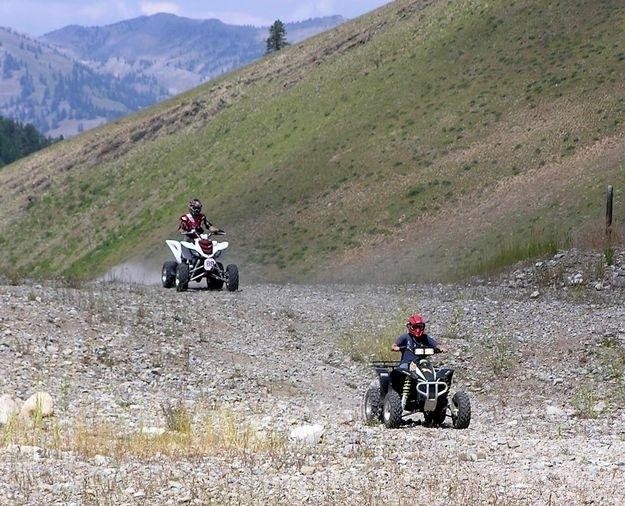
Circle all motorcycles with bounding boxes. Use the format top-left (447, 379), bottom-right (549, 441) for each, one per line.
top-left (361, 345), bottom-right (471, 430)
top-left (160, 225), bottom-right (240, 292)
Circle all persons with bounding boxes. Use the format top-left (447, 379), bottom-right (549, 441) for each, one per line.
top-left (176, 197), bottom-right (220, 264)
top-left (390, 315), bottom-right (448, 397)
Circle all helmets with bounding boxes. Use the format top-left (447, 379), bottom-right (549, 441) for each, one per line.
top-left (406, 314), bottom-right (425, 337)
top-left (188, 199), bottom-right (202, 216)
